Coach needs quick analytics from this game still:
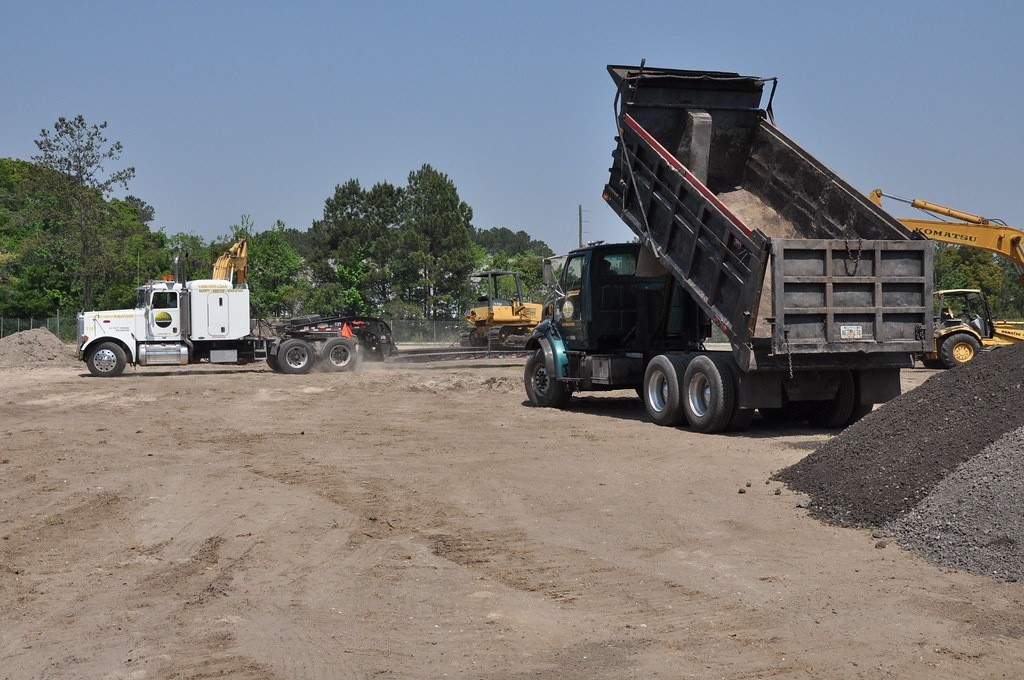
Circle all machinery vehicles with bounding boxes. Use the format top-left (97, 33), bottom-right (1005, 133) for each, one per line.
top-left (73, 254), bottom-right (393, 374)
top-left (461, 271), bottom-right (543, 350)
top-left (521, 60), bottom-right (937, 434)
top-left (211, 236), bottom-right (255, 294)
top-left (869, 187), bottom-right (1024, 369)
